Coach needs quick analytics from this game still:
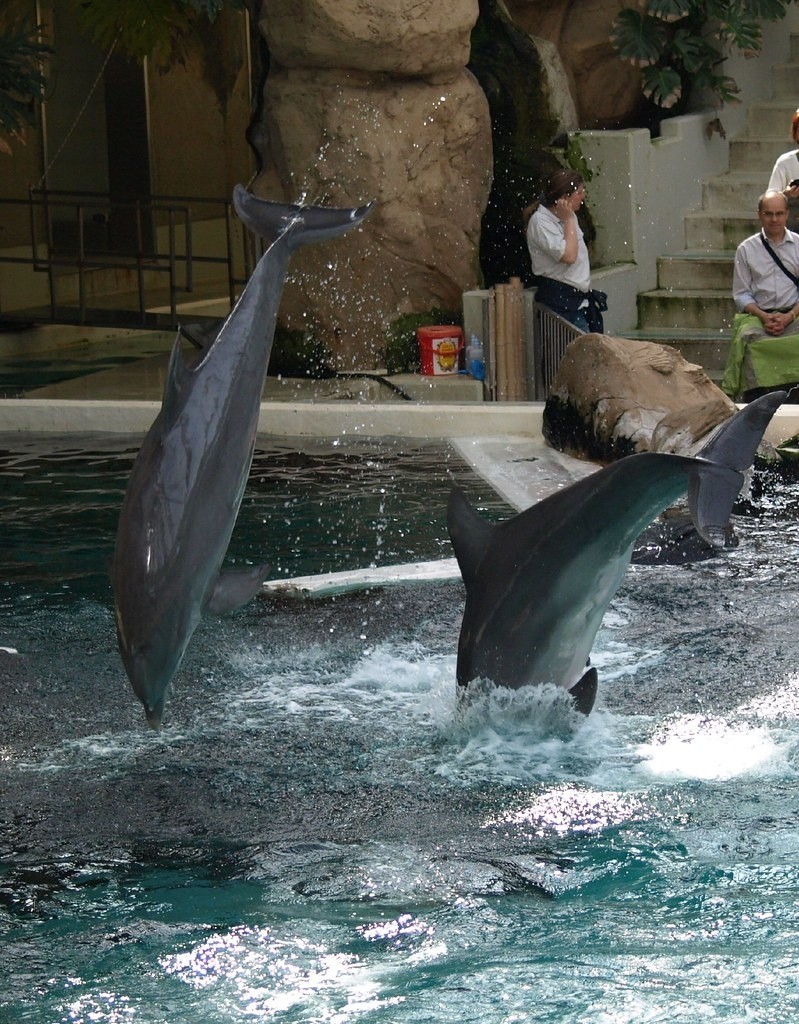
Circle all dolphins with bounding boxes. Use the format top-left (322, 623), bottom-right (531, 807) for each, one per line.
top-left (444, 387), bottom-right (792, 746)
top-left (110, 183), bottom-right (378, 733)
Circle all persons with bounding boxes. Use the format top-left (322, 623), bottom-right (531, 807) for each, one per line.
top-left (721, 189), bottom-right (799, 402)
top-left (768, 109), bottom-right (799, 235)
top-left (524, 169), bottom-right (591, 389)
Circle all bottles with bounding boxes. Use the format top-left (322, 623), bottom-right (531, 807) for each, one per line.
top-left (470, 338), bottom-right (486, 380)
top-left (465, 333), bottom-right (476, 373)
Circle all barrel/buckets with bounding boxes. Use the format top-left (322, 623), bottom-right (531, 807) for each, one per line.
top-left (417, 325), bottom-right (464, 376)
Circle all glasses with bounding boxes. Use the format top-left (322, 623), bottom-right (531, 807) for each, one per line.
top-left (759, 210), bottom-right (785, 218)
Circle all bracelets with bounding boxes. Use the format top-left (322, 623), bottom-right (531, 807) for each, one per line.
top-left (790, 310), bottom-right (795, 320)
